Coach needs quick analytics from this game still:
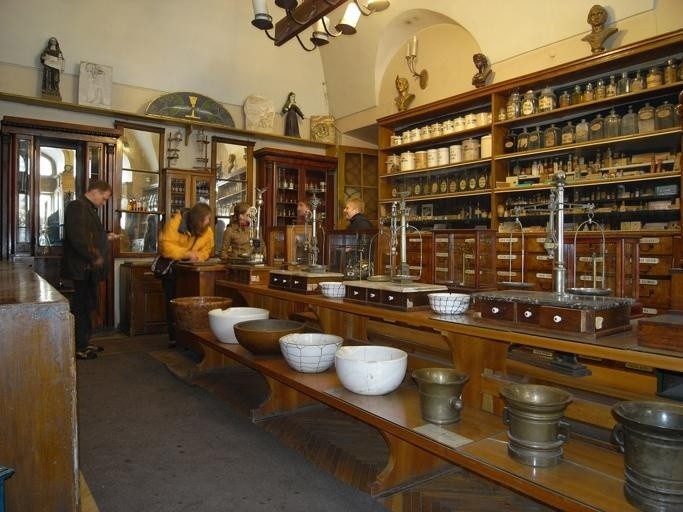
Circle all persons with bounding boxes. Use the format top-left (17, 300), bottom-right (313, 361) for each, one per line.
top-left (156, 203), bottom-right (217, 345)
top-left (337, 194), bottom-right (376, 266)
top-left (394, 75), bottom-right (415, 111)
top-left (469, 54), bottom-right (493, 90)
top-left (280, 90), bottom-right (307, 138)
top-left (59, 180), bottom-right (112, 360)
top-left (296, 198), bottom-right (314, 227)
top-left (39, 37), bottom-right (64, 96)
top-left (580, 5), bottom-right (620, 54)
top-left (226, 154), bottom-right (237, 174)
top-left (221, 202), bottom-right (267, 263)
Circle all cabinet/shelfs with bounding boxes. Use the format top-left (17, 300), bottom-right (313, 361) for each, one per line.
top-left (119, 264), bottom-right (174, 335)
top-left (267, 269), bottom-right (345, 296)
top-left (375, 30), bottom-right (683, 309)
top-left (253, 147), bottom-right (338, 265)
top-left (165, 169), bottom-right (216, 226)
top-left (137, 183), bottom-right (158, 239)
top-left (342, 278), bottom-right (448, 313)
top-left (473, 289), bottom-right (633, 339)
top-left (217, 167), bottom-right (247, 215)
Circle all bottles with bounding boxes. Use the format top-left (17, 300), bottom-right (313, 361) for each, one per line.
top-left (386, 134), bottom-right (492, 175)
top-left (277, 167), bottom-right (326, 219)
top-left (127, 193), bottom-right (158, 212)
top-left (496, 57), bottom-right (683, 218)
top-left (390, 111), bottom-right (491, 146)
top-left (390, 164), bottom-right (492, 229)
top-left (171, 179), bottom-right (209, 214)
top-left (216, 181), bottom-right (243, 216)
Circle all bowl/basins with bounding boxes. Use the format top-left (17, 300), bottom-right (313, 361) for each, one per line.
top-left (170, 295), bottom-right (232, 328)
top-left (234, 320), bottom-right (308, 357)
top-left (427, 293), bottom-right (471, 315)
top-left (279, 332), bottom-right (343, 374)
top-left (335, 346), bottom-right (407, 397)
top-left (318, 281), bottom-right (352, 299)
top-left (208, 305), bottom-right (268, 344)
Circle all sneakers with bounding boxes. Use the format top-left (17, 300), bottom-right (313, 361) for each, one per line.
top-left (76, 345), bottom-right (104, 360)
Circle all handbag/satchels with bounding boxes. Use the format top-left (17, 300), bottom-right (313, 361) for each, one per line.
top-left (151, 255), bottom-right (175, 278)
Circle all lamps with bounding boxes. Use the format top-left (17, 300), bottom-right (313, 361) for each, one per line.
top-left (405, 35), bottom-right (429, 89)
top-left (251, 0), bottom-right (390, 52)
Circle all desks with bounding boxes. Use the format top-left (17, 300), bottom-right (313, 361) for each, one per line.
top-left (187, 325), bottom-right (639, 512)
top-left (0, 261), bottom-right (79, 511)
